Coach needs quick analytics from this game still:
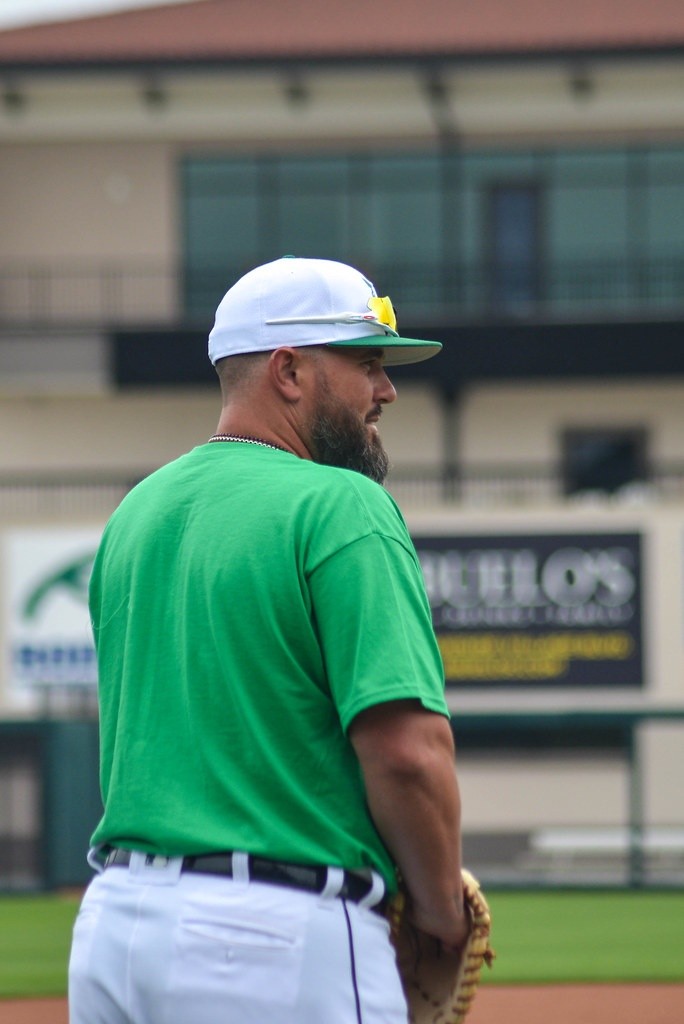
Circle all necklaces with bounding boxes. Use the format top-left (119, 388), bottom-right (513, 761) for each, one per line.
top-left (208, 433), bottom-right (292, 454)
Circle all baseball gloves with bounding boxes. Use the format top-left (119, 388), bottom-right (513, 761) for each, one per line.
top-left (391, 865), bottom-right (495, 1022)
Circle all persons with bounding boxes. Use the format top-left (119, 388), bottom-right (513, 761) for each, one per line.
top-left (61, 258), bottom-right (470, 1024)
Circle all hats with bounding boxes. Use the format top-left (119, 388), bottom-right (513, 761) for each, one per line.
top-left (207, 254), bottom-right (442, 366)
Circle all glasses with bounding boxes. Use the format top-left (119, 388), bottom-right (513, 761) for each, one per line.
top-left (264, 297), bottom-right (397, 336)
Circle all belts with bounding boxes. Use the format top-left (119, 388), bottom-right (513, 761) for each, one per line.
top-left (103, 850), bottom-right (392, 917)
